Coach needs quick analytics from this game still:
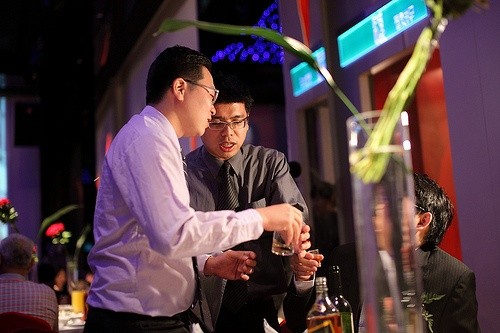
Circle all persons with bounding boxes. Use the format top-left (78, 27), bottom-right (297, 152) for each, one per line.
top-left (51, 268), bottom-right (71, 304)
top-left (186, 86), bottom-right (310, 333)
top-left (0, 234), bottom-right (57, 331)
top-left (83, 47), bottom-right (302, 332)
top-left (283, 174), bottom-right (482, 333)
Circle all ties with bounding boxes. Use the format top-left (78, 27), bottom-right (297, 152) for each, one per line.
top-left (219, 162), bottom-right (249, 310)
top-left (180, 151), bottom-right (213, 330)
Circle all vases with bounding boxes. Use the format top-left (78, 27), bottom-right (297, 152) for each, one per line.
top-left (66, 264), bottom-right (79, 295)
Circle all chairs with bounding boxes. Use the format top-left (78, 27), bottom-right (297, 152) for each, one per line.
top-left (0, 312), bottom-right (53, 333)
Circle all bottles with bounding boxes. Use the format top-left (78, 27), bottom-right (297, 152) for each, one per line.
top-left (307, 277), bottom-right (343, 333)
top-left (330, 265), bottom-right (354, 333)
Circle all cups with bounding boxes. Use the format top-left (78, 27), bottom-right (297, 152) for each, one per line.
top-left (272, 231), bottom-right (294, 256)
top-left (71, 281), bottom-right (90, 321)
top-left (308, 249), bottom-right (320, 267)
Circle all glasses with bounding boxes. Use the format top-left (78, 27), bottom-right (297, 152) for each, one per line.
top-left (169, 77), bottom-right (219, 104)
top-left (208, 117), bottom-right (248, 130)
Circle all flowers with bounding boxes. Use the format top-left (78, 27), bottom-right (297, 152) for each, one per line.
top-left (45, 221), bottom-right (74, 263)
top-left (0, 197), bottom-right (19, 233)
top-left (32, 244), bottom-right (39, 263)
